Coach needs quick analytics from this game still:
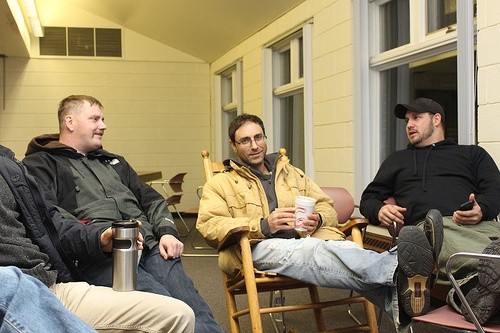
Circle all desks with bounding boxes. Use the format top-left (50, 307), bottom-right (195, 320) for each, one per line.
top-left (137, 171), bottom-right (162, 182)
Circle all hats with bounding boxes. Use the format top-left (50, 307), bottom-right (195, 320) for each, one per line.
top-left (394, 97), bottom-right (445, 123)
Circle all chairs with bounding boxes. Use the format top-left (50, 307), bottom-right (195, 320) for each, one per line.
top-left (150, 150), bottom-right (500, 333)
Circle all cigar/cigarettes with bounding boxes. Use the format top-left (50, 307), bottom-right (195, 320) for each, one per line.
top-left (392, 221), bottom-right (397, 228)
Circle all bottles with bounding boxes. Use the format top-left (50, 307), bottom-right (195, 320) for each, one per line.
top-left (273, 290), bottom-right (285, 321)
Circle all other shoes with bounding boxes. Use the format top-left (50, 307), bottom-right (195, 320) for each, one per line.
top-left (424, 209), bottom-right (444, 288)
top-left (397, 225), bottom-right (435, 318)
top-left (461, 239), bottom-right (500, 326)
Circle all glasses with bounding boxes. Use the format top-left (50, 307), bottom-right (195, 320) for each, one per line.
top-left (232, 135), bottom-right (267, 145)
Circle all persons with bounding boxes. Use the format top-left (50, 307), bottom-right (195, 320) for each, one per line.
top-left (359, 97), bottom-right (500, 325)
top-left (21, 96), bottom-right (225, 333)
top-left (0, 144), bottom-right (196, 333)
top-left (195, 111), bottom-right (445, 332)
top-left (0, 265), bottom-right (98, 333)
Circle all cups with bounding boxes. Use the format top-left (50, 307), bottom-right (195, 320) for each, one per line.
top-left (293, 195), bottom-right (317, 232)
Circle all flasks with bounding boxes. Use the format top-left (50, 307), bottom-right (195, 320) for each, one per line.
top-left (112, 220), bottom-right (138, 293)
